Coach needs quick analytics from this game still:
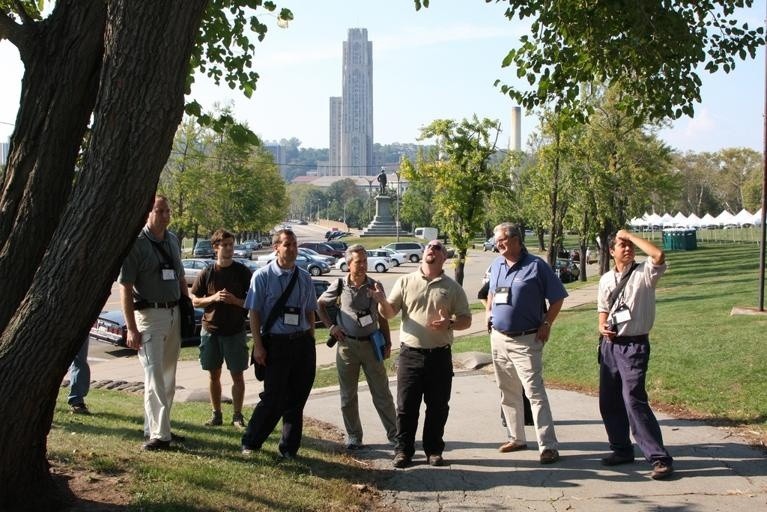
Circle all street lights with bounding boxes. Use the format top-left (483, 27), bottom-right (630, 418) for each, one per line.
top-left (394, 170), bottom-right (401, 241)
top-left (305, 199), bottom-right (348, 226)
top-left (360, 175), bottom-right (378, 220)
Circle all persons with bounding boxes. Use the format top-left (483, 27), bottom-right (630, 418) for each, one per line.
top-left (364, 240), bottom-right (472, 467)
top-left (477, 223), bottom-right (569, 464)
top-left (572, 246), bottom-right (591, 264)
top-left (316, 245), bottom-right (397, 451)
top-left (67, 335), bottom-right (92, 413)
top-left (597, 229), bottom-right (673, 479)
top-left (377, 170), bottom-right (386, 193)
top-left (188, 229), bottom-right (253, 429)
top-left (116, 194), bottom-right (190, 451)
top-left (241, 229), bottom-right (318, 459)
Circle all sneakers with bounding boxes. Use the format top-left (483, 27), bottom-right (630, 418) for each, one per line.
top-left (232, 415), bottom-right (244, 426)
top-left (205, 410), bottom-right (222, 426)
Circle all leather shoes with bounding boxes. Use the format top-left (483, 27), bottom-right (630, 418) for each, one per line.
top-left (500, 442), bottom-right (526, 451)
top-left (541, 449), bottom-right (559, 463)
top-left (652, 465), bottom-right (673, 479)
top-left (392, 453), bottom-right (411, 467)
top-left (601, 453), bottom-right (634, 465)
top-left (427, 454), bottom-right (442, 465)
top-left (143, 438), bottom-right (170, 450)
top-left (73, 403), bottom-right (87, 413)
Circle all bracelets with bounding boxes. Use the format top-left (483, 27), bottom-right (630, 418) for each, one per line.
top-left (328, 324), bottom-right (335, 332)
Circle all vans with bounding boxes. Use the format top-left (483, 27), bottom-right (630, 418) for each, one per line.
top-left (337, 249), bottom-right (391, 273)
top-left (414, 227), bottom-right (438, 242)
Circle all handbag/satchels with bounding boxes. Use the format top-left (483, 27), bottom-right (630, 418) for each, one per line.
top-left (180, 296), bottom-right (196, 338)
top-left (201, 308), bottom-right (236, 336)
top-left (250, 344), bottom-right (264, 381)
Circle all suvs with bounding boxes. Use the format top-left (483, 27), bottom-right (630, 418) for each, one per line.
top-left (381, 242), bottom-right (424, 262)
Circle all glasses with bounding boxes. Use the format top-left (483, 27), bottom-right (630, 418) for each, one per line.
top-left (426, 245), bottom-right (441, 250)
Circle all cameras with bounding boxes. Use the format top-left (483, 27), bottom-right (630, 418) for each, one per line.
top-left (606, 319), bottom-right (617, 333)
top-left (327, 330), bottom-right (344, 348)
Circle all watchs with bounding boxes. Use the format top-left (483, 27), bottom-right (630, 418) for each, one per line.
top-left (448, 319), bottom-right (455, 331)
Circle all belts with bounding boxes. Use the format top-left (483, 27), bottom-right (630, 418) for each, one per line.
top-left (133, 302), bottom-right (179, 310)
top-left (504, 330), bottom-right (537, 337)
top-left (348, 335), bottom-right (371, 340)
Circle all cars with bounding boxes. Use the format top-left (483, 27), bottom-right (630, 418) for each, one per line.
top-left (554, 259), bottom-right (579, 281)
top-left (524, 230), bottom-right (536, 236)
top-left (482, 236), bottom-right (498, 253)
top-left (89, 211), bottom-right (351, 348)
top-left (559, 246), bottom-right (599, 264)
top-left (380, 249), bottom-right (407, 267)
